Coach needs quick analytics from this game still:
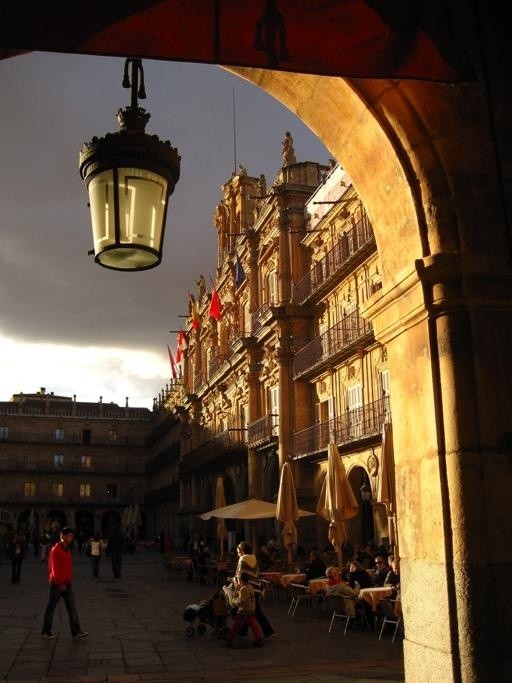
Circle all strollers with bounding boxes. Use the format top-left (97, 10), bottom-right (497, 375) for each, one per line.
top-left (183, 577), bottom-right (234, 642)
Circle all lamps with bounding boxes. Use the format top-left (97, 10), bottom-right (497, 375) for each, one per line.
top-left (360, 471), bottom-right (371, 502)
top-left (77, 54), bottom-right (181, 271)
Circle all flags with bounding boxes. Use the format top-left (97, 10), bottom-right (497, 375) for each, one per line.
top-left (233, 255), bottom-right (246, 284)
top-left (167, 346), bottom-right (178, 381)
top-left (176, 333), bottom-right (187, 356)
top-left (191, 317), bottom-right (198, 332)
top-left (210, 291), bottom-right (222, 320)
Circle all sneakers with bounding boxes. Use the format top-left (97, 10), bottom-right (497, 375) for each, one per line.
top-left (41, 631), bottom-right (54, 639)
top-left (75, 631), bottom-right (88, 640)
top-left (265, 632), bottom-right (279, 642)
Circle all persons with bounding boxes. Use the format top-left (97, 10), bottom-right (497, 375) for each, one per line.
top-left (40, 526), bottom-right (89, 641)
top-left (282, 131), bottom-right (293, 166)
top-left (1, 518), bottom-right (162, 584)
top-left (188, 293), bottom-right (196, 315)
top-left (238, 164), bottom-right (247, 175)
top-left (196, 274), bottom-right (207, 295)
top-left (162, 519), bottom-right (401, 649)
top-left (327, 158), bottom-right (336, 170)
top-left (257, 174), bottom-right (266, 197)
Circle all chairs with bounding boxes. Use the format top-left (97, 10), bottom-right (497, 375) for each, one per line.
top-left (158, 540), bottom-right (403, 644)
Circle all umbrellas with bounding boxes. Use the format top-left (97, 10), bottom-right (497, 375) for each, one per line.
top-left (377, 394), bottom-right (399, 560)
top-left (316, 442), bottom-right (359, 566)
top-left (127, 505), bottom-right (134, 531)
top-left (120, 505), bottom-right (128, 538)
top-left (132, 504), bottom-right (143, 530)
top-left (193, 498), bottom-right (317, 556)
top-left (275, 462), bottom-right (301, 562)
top-left (215, 476), bottom-right (228, 558)
